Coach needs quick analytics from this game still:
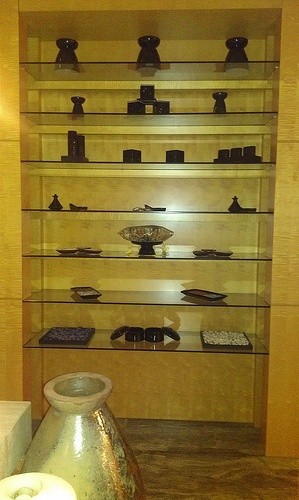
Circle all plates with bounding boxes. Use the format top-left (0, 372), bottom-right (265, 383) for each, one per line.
top-left (182, 288), bottom-right (228, 302)
top-left (193, 248), bottom-right (233, 257)
top-left (70, 285), bottom-right (102, 299)
top-left (55, 245), bottom-right (104, 255)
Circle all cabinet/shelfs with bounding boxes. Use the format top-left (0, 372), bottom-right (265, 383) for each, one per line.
top-left (18, 50), bottom-right (280, 356)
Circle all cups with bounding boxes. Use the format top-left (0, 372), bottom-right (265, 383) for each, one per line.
top-left (217, 145), bottom-right (256, 157)
